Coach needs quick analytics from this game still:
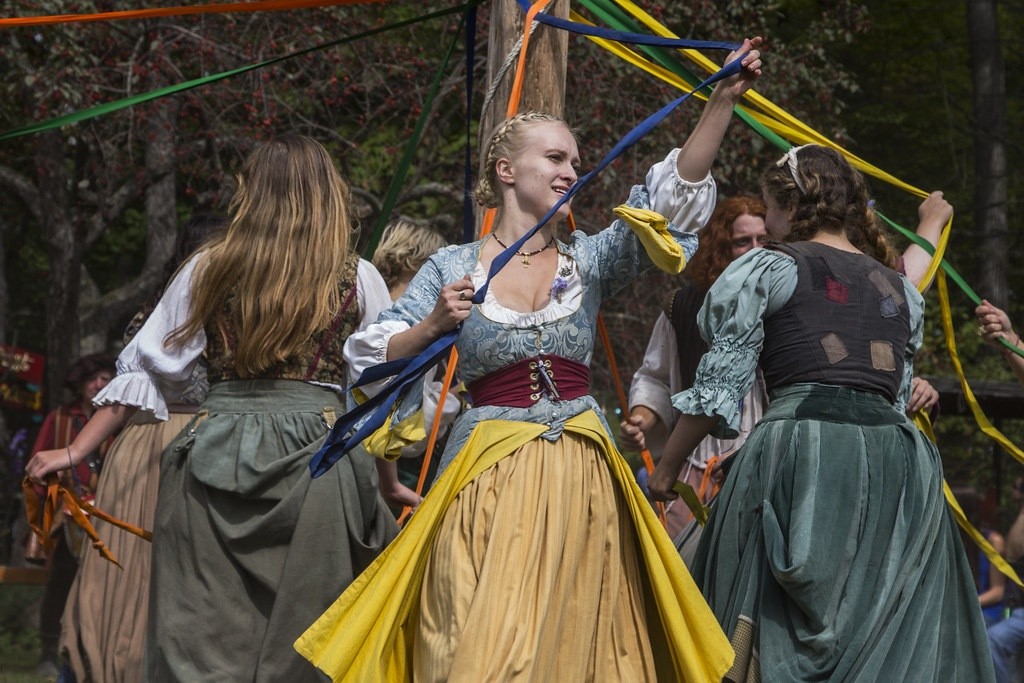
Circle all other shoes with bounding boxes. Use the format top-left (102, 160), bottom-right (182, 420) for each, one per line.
top-left (55, 662), bottom-right (76, 683)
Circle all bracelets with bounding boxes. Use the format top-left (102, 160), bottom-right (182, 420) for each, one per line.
top-left (1007, 334), bottom-right (1019, 357)
top-left (67, 446), bottom-right (73, 469)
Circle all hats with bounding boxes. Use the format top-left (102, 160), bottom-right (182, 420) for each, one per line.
top-left (64, 354), bottom-right (118, 391)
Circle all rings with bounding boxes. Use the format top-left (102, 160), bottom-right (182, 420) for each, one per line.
top-left (460, 290), bottom-right (465, 300)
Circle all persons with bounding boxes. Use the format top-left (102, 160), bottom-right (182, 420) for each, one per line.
top-left (618, 194), bottom-right (939, 571)
top-left (951, 300), bottom-right (1024, 683)
top-left (291, 37), bottom-right (763, 683)
top-left (57, 213), bottom-right (234, 683)
top-left (0, 353), bottom-right (117, 675)
top-left (348, 215), bottom-right (471, 528)
top-left (647, 143), bottom-right (996, 683)
top-left (24, 132), bottom-right (425, 683)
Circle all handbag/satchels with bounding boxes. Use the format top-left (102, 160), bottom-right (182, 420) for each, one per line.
top-left (25, 518), bottom-right (64, 566)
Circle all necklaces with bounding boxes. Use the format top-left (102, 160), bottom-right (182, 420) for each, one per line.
top-left (493, 234), bottom-right (553, 268)
